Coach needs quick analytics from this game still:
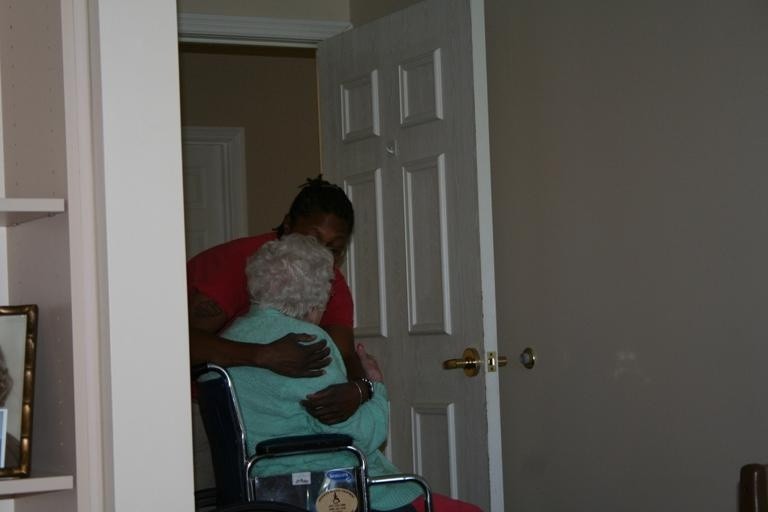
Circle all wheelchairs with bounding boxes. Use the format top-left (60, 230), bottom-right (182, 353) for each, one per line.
top-left (190, 362), bottom-right (431, 511)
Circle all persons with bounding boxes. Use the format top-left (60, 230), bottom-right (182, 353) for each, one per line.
top-left (204, 226), bottom-right (492, 512)
top-left (180, 171), bottom-right (374, 512)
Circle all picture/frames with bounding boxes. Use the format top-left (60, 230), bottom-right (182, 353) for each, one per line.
top-left (1, 305), bottom-right (39, 476)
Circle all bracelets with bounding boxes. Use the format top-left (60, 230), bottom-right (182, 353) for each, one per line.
top-left (352, 381), bottom-right (364, 405)
top-left (358, 377), bottom-right (373, 400)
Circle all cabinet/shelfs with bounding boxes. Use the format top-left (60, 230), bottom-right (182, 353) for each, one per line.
top-left (1, 196), bottom-right (73, 498)
